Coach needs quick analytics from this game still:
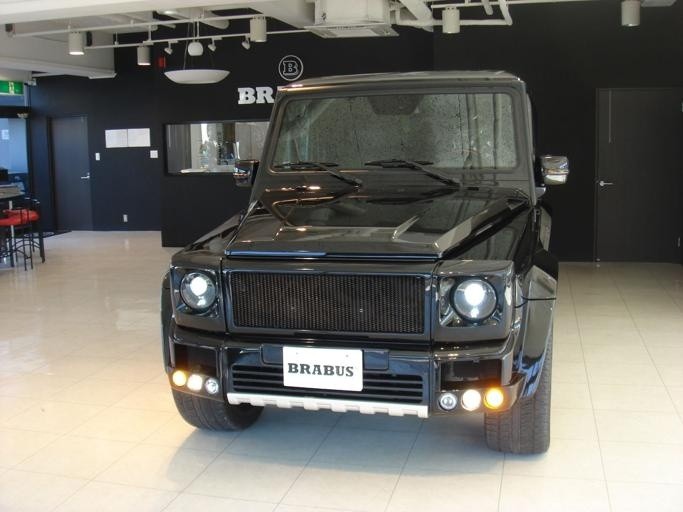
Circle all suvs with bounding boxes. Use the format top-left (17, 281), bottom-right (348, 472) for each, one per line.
top-left (160, 68), bottom-right (571, 457)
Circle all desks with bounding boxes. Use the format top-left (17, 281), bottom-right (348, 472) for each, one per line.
top-left (0, 184), bottom-right (24, 264)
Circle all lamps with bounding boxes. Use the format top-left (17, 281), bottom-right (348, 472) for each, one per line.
top-left (440, 5), bottom-right (461, 36)
top-left (66, 12), bottom-right (269, 87)
top-left (619, 0), bottom-right (642, 29)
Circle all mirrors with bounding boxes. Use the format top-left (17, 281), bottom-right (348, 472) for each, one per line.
top-left (161, 117), bottom-right (270, 174)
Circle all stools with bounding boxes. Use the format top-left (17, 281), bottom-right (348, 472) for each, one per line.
top-left (0, 195), bottom-right (44, 272)
